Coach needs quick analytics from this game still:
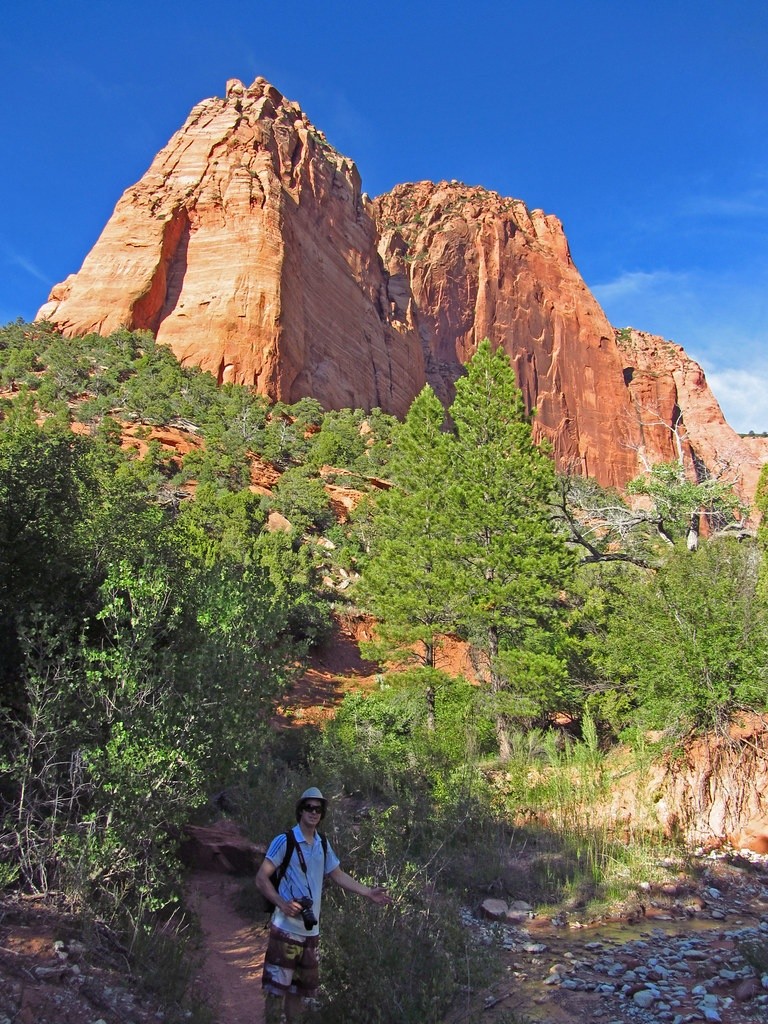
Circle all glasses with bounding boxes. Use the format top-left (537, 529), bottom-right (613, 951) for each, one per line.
top-left (299, 804), bottom-right (324, 814)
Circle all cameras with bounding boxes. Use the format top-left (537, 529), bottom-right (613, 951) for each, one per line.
top-left (294, 896), bottom-right (318, 931)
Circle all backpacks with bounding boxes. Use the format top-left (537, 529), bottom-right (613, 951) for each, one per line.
top-left (261, 829), bottom-right (328, 914)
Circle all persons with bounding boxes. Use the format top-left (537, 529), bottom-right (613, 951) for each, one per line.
top-left (255, 786), bottom-right (397, 1023)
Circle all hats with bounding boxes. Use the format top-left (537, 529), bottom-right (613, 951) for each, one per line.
top-left (295, 787), bottom-right (328, 808)
